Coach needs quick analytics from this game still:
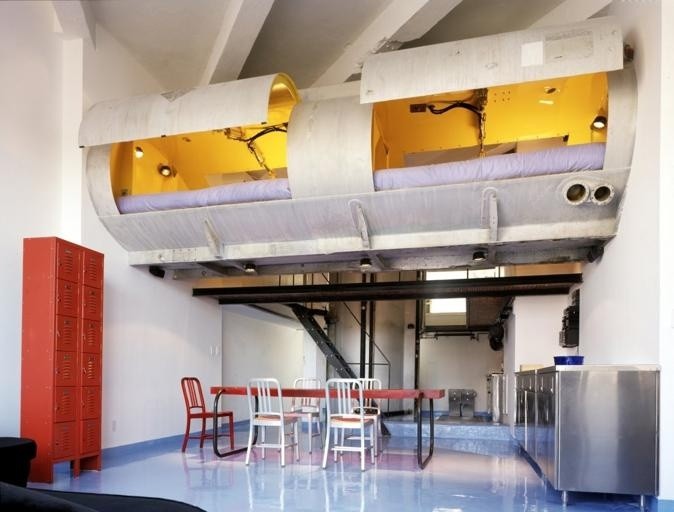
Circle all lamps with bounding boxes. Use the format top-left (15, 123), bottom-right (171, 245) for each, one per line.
top-left (245, 263), bottom-right (256, 272)
top-left (159, 166), bottom-right (172, 176)
top-left (360, 258), bottom-right (371, 267)
top-left (590, 116), bottom-right (607, 132)
top-left (473, 251), bottom-right (485, 260)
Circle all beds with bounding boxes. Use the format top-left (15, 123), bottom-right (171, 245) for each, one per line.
top-left (117, 176), bottom-right (291, 214)
top-left (374, 141), bottom-right (604, 189)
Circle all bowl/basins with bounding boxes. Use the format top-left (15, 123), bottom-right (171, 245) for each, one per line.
top-left (554, 355), bottom-right (583, 365)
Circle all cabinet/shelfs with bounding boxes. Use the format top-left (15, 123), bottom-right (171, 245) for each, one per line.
top-left (515, 372), bottom-right (659, 497)
top-left (21, 237), bottom-right (104, 483)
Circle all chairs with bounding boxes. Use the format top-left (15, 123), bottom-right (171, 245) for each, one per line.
top-left (245, 378), bottom-right (382, 471)
top-left (181, 377), bottom-right (234, 452)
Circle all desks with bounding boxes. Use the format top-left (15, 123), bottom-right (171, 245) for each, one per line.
top-left (211, 387), bottom-right (445, 470)
